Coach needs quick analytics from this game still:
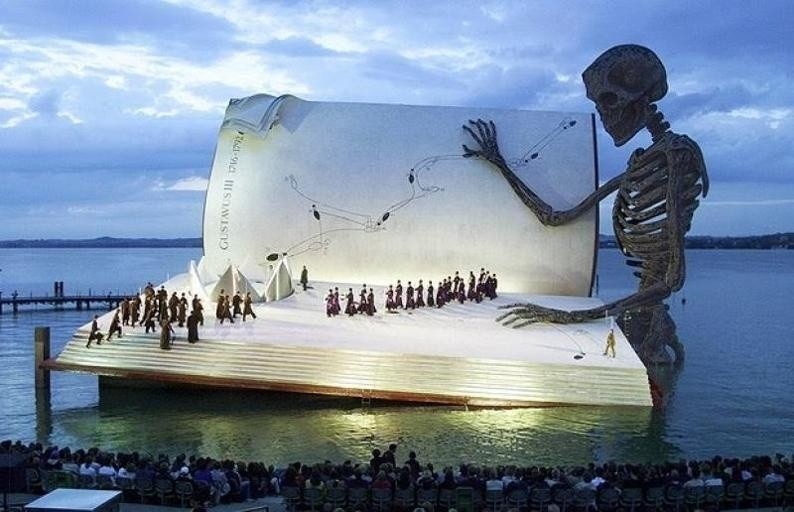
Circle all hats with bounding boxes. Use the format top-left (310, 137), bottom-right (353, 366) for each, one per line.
top-left (356, 463), bottom-right (433, 478)
top-left (180, 467), bottom-right (189, 474)
top-left (51, 451), bottom-right (59, 459)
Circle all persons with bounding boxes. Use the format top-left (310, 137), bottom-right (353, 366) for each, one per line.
top-left (603, 329), bottom-right (616, 357)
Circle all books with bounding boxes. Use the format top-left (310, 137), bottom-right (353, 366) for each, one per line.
top-left (38, 93), bottom-right (655, 407)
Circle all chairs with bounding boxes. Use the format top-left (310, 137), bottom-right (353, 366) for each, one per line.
top-left (22, 464), bottom-right (792, 510)
